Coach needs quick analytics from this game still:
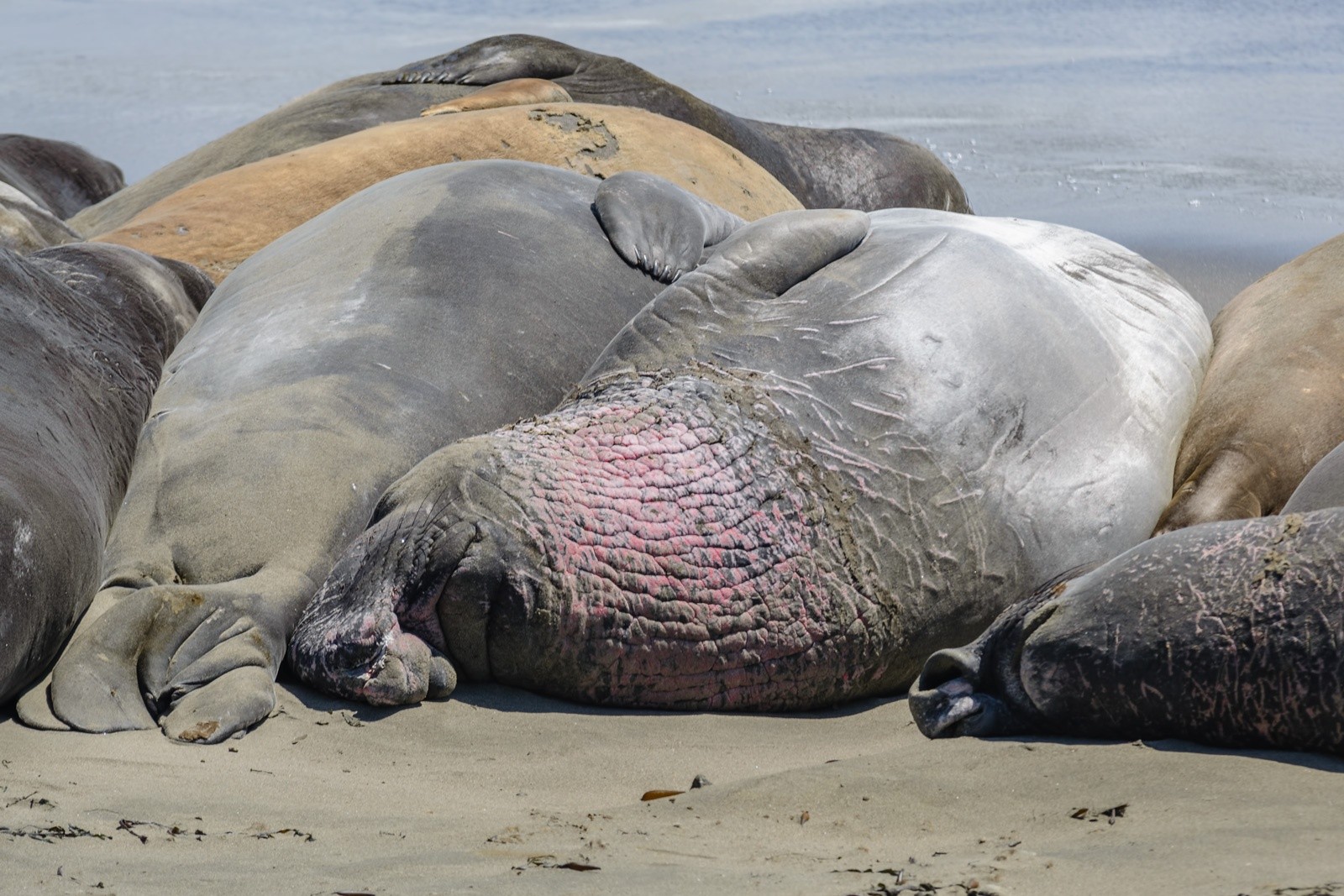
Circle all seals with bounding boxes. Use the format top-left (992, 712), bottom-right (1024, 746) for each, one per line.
top-left (0, 33), bottom-right (1344, 761)
